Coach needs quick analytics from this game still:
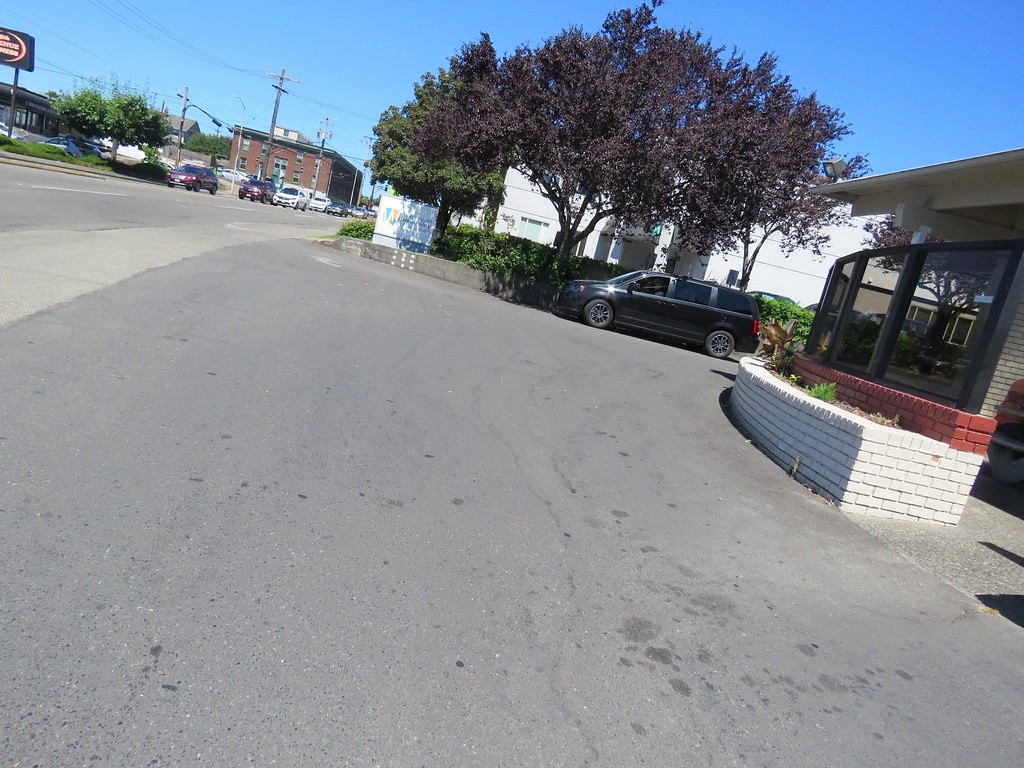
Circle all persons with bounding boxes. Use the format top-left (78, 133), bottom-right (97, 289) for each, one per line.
top-left (649, 279), bottom-right (669, 298)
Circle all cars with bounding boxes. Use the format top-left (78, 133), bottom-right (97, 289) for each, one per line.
top-left (36, 137), bottom-right (102, 159)
top-left (867, 314), bottom-right (930, 337)
top-left (803, 303), bottom-right (869, 322)
top-left (221, 168), bottom-right (377, 219)
top-left (564, 270), bottom-right (761, 358)
top-left (166, 163), bottom-right (218, 195)
top-left (747, 291), bottom-right (796, 306)
top-left (0, 124), bottom-right (25, 142)
